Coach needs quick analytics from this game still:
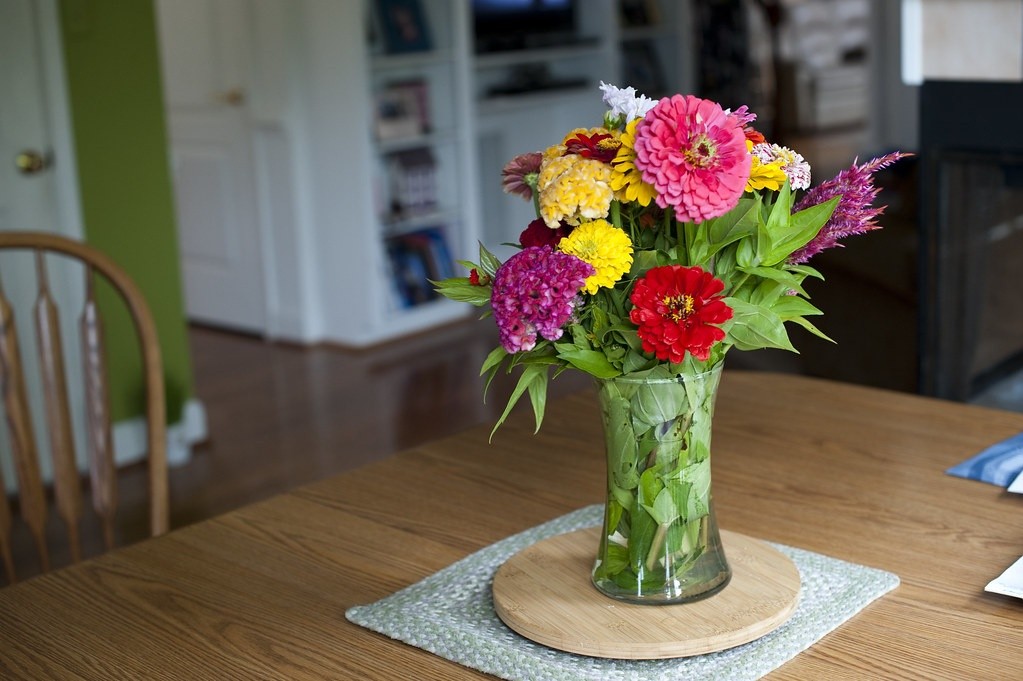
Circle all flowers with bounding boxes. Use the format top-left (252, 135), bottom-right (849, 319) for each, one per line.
top-left (426, 81), bottom-right (916, 586)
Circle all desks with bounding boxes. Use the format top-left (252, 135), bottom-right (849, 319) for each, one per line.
top-left (0, 368), bottom-right (1023, 681)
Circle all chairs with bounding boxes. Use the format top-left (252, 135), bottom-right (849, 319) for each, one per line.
top-left (0, 230), bottom-right (171, 587)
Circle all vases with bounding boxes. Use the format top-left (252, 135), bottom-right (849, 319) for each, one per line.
top-left (596, 365), bottom-right (740, 604)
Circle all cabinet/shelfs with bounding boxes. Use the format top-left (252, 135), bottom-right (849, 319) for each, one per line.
top-left (359, 1), bottom-right (695, 341)
